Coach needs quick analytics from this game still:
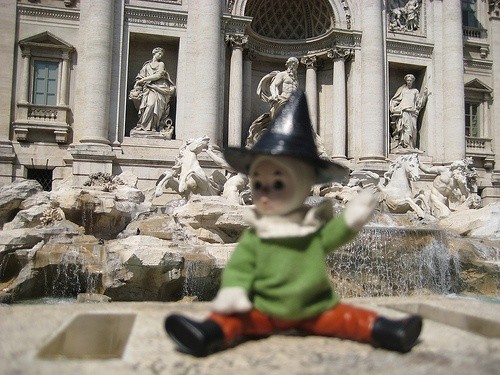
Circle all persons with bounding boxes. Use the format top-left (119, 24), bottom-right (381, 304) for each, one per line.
top-left (134, 47), bottom-right (171, 132)
top-left (270, 57), bottom-right (299, 119)
top-left (165, 151), bottom-right (425, 357)
top-left (203, 143), bottom-right (249, 205)
top-left (389, 73), bottom-right (428, 149)
top-left (417, 160), bottom-right (471, 220)
top-left (404, 0), bottom-right (419, 31)
